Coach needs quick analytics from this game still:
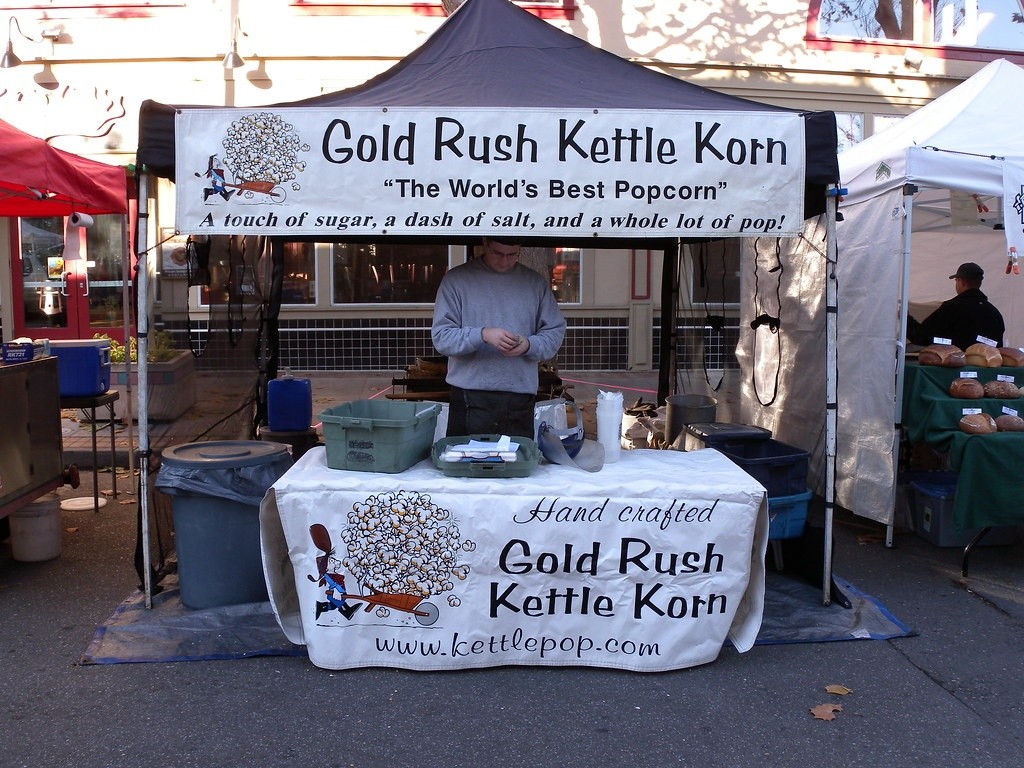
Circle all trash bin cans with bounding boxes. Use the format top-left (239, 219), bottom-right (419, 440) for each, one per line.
top-left (161, 440), bottom-right (291, 612)
top-left (664, 394), bottom-right (719, 446)
top-left (682, 422), bottom-right (772, 452)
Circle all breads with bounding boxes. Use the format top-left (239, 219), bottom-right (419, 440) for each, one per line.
top-left (995, 414), bottom-right (1024, 431)
top-left (983, 380), bottom-right (1020, 398)
top-left (959, 413), bottom-right (998, 433)
top-left (998, 346), bottom-right (1024, 367)
top-left (1018, 386), bottom-right (1024, 395)
top-left (950, 378), bottom-right (984, 399)
top-left (965, 343), bottom-right (1003, 367)
top-left (917, 344), bottom-right (967, 367)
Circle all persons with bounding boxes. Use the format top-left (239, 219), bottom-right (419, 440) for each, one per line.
top-left (430, 237), bottom-right (567, 441)
top-left (907, 262), bottom-right (1005, 351)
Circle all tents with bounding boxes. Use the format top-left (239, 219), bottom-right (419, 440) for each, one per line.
top-left (137, 0), bottom-right (840, 606)
top-left (736, 57), bottom-right (1024, 549)
top-left (1, 120), bottom-right (135, 493)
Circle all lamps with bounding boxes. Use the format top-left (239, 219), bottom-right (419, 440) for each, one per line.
top-left (0, 15), bottom-right (43, 68)
top-left (221, 16), bottom-right (248, 68)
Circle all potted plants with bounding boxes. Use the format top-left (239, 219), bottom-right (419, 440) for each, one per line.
top-left (78, 332), bottom-right (198, 423)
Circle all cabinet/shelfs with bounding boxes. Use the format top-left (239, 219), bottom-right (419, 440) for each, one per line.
top-left (905, 362), bottom-right (1024, 577)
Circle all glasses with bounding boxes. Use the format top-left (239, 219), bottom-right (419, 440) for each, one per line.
top-left (485, 239), bottom-right (521, 261)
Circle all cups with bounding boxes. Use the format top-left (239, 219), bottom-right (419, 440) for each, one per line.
top-left (596, 392), bottom-right (624, 464)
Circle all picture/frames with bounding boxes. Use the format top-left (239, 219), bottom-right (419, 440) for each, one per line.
top-left (46, 256), bottom-right (65, 280)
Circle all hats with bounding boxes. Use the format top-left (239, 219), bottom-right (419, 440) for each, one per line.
top-left (538, 400), bottom-right (606, 472)
top-left (950, 263), bottom-right (985, 282)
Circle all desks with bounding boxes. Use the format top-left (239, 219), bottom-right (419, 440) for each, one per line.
top-left (60, 389), bottom-right (120, 513)
top-left (0, 355), bottom-right (81, 517)
top-left (258, 447), bottom-right (768, 672)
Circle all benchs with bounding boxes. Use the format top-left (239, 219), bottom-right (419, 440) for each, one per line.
top-left (385, 377), bottom-right (449, 401)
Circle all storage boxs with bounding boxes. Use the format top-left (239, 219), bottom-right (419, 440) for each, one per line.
top-left (318, 399), bottom-right (442, 474)
top-left (682, 422), bottom-right (773, 452)
top-left (2, 338), bottom-right (51, 363)
top-left (768, 490), bottom-right (812, 539)
top-left (49, 339), bottom-right (111, 397)
top-left (911, 471), bottom-right (1024, 546)
top-left (706, 438), bottom-right (811, 494)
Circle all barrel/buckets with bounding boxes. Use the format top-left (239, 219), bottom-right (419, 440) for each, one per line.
top-left (664, 393), bottom-right (719, 446)
top-left (159, 439), bottom-right (295, 611)
top-left (9, 492), bottom-right (63, 561)
top-left (267, 368), bottom-right (312, 432)
